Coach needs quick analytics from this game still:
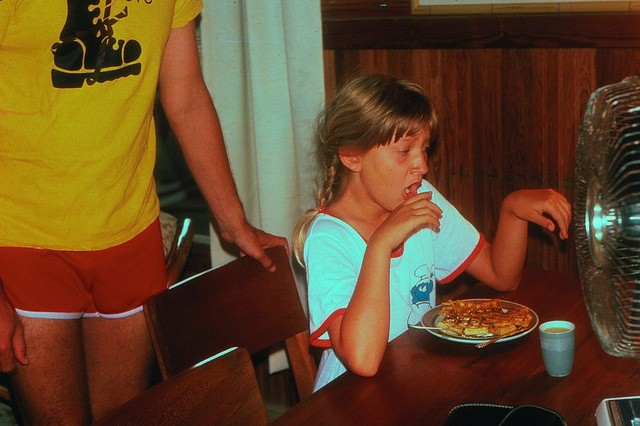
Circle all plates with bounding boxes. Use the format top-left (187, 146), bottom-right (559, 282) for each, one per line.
top-left (420, 298), bottom-right (539, 344)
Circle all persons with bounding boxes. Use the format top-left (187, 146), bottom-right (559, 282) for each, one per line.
top-left (293, 73), bottom-right (573, 393)
top-left (0, 0), bottom-right (290, 423)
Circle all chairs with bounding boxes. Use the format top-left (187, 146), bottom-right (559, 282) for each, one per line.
top-left (82, 347), bottom-right (270, 425)
top-left (143, 246), bottom-right (317, 403)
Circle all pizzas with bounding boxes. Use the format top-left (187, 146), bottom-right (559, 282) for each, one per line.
top-left (438, 298), bottom-right (530, 335)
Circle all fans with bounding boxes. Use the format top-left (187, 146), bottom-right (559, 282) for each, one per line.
top-left (571, 76), bottom-right (640, 426)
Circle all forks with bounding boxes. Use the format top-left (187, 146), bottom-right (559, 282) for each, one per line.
top-left (409, 325), bottom-right (486, 339)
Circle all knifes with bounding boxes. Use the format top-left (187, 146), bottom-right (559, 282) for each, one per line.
top-left (474, 327), bottom-right (530, 349)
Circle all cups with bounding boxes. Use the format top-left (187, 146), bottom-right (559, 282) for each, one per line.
top-left (539, 320), bottom-right (575, 377)
